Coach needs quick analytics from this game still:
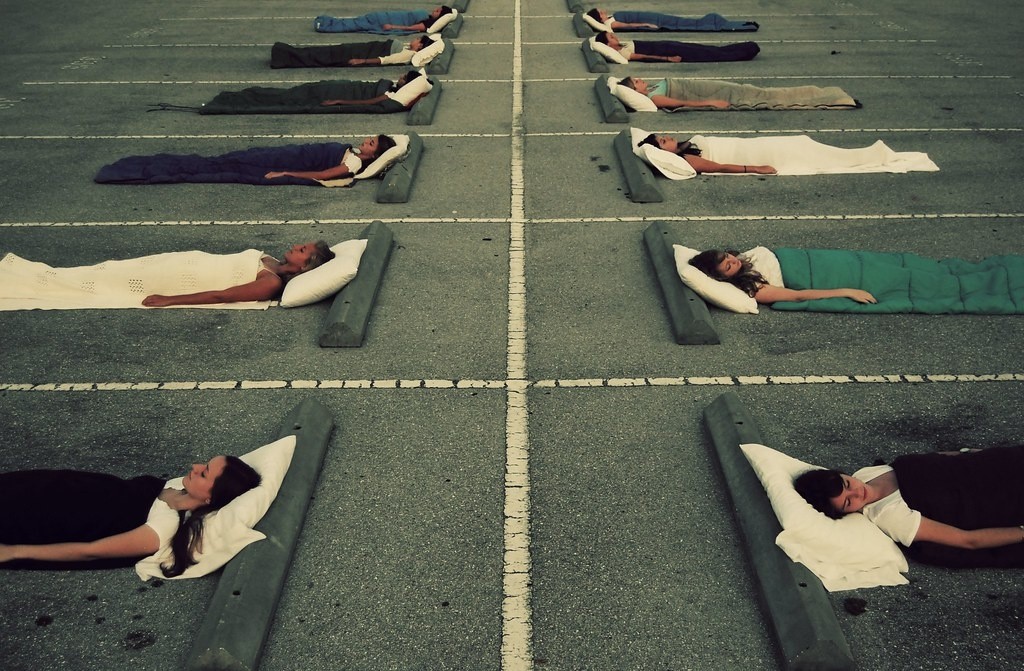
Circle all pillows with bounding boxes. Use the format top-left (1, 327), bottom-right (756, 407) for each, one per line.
top-left (411, 33), bottom-right (445, 67)
top-left (606, 76), bottom-right (658, 112)
top-left (281, 238), bottom-right (368, 308)
top-left (739, 444), bottom-right (910, 592)
top-left (630, 127), bottom-right (697, 180)
top-left (673, 244), bottom-right (759, 314)
top-left (393, 68), bottom-right (433, 106)
top-left (427, 8), bottom-right (458, 34)
top-left (354, 134), bottom-right (410, 179)
top-left (583, 13), bottom-right (613, 33)
top-left (135, 436), bottom-right (297, 581)
top-left (589, 36), bottom-right (628, 65)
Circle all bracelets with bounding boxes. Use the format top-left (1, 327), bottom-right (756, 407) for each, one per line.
top-left (666, 56), bottom-right (669, 62)
top-left (960, 448), bottom-right (971, 454)
top-left (744, 165), bottom-right (747, 173)
top-left (1019, 525), bottom-right (1024, 531)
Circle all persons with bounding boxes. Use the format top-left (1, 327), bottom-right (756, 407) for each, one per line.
top-left (264, 135), bottom-right (396, 179)
top-left (617, 76), bottom-right (729, 108)
top-left (793, 448), bottom-right (1024, 549)
top-left (637, 133), bottom-right (777, 174)
top-left (0, 456), bottom-right (261, 577)
top-left (348, 4), bottom-right (452, 65)
top-left (142, 240), bottom-right (335, 306)
top-left (586, 7), bottom-right (681, 63)
top-left (321, 71), bottom-right (434, 107)
top-left (689, 246), bottom-right (877, 305)
